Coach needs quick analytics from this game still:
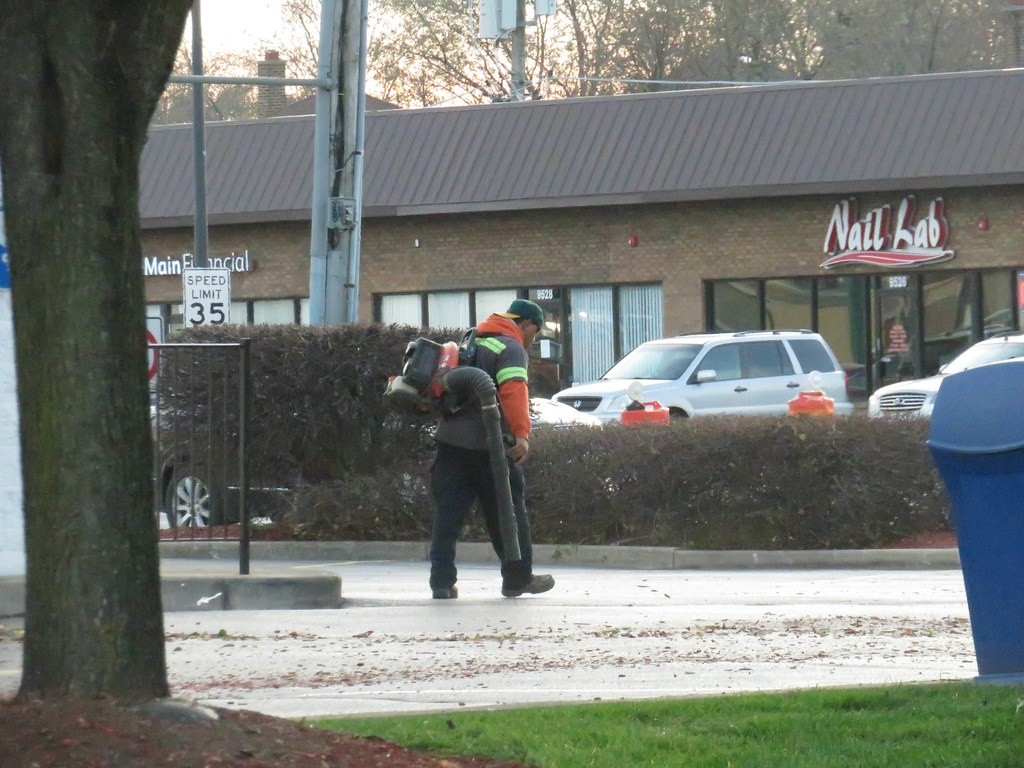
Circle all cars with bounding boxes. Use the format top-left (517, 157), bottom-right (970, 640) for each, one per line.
top-left (149, 389), bottom-right (615, 534)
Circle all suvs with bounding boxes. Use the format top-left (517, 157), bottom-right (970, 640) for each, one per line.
top-left (865, 331), bottom-right (1023, 421)
top-left (548, 327), bottom-right (855, 430)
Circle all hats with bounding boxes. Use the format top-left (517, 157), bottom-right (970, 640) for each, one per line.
top-left (492, 298), bottom-right (543, 330)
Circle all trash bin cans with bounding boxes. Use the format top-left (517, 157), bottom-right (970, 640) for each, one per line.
top-left (924, 334), bottom-right (1024, 685)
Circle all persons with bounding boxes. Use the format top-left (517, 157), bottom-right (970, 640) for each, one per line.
top-left (424, 297), bottom-right (557, 596)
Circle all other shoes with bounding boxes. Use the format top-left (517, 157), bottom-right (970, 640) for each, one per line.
top-left (503, 575), bottom-right (554, 599)
top-left (433, 585), bottom-right (459, 599)
